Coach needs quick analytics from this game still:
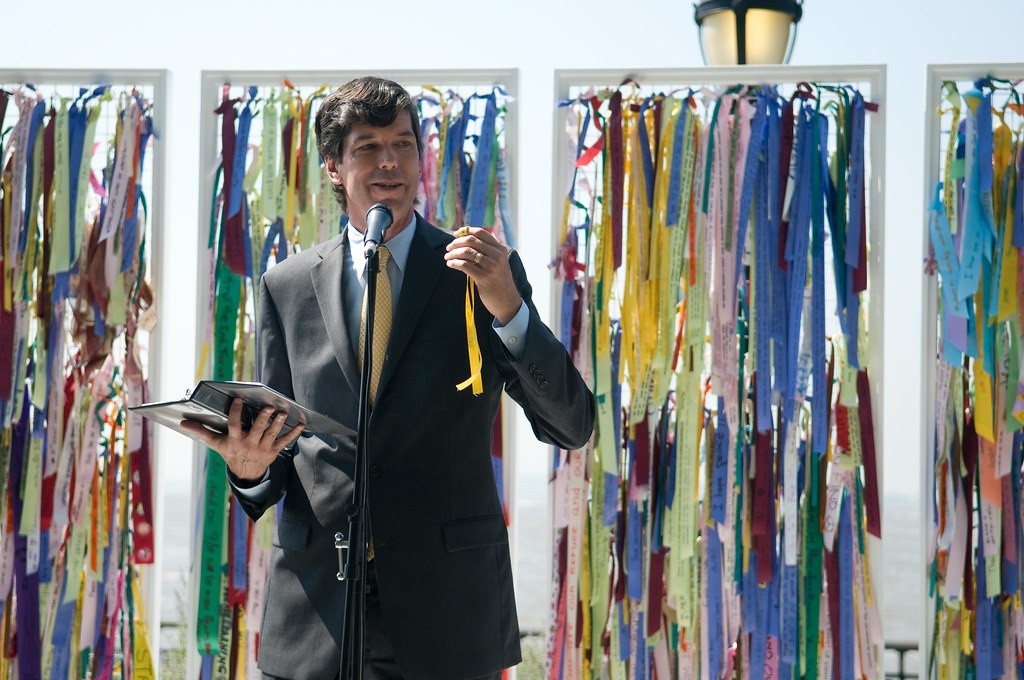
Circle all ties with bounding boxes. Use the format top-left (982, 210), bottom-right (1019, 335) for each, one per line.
top-left (357, 247), bottom-right (393, 412)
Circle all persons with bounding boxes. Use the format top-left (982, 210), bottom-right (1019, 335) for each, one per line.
top-left (177, 74), bottom-right (596, 680)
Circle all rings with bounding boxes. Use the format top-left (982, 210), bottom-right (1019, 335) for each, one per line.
top-left (474, 253), bottom-right (483, 265)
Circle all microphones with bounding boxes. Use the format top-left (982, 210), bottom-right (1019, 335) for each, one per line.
top-left (364, 202), bottom-right (394, 261)
